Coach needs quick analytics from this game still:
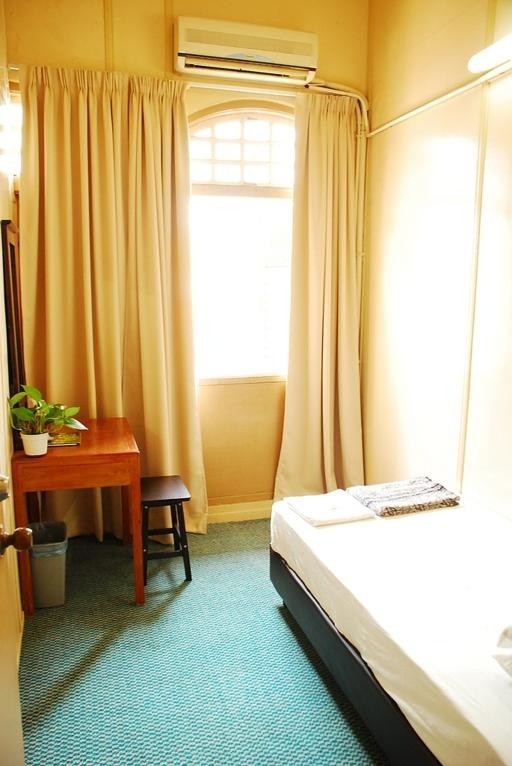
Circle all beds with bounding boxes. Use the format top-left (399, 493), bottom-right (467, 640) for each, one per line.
top-left (269, 481), bottom-right (512, 766)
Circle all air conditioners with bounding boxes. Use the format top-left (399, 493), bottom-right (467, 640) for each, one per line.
top-left (172, 13), bottom-right (320, 88)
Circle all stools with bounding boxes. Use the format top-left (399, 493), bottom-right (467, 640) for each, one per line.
top-left (141, 475), bottom-right (195, 586)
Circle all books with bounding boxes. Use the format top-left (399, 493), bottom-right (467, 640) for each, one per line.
top-left (48, 431), bottom-right (82, 447)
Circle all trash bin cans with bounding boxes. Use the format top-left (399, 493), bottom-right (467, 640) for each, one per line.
top-left (26, 519), bottom-right (68, 609)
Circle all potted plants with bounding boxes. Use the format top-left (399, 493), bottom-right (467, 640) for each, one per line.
top-left (7, 384), bottom-right (89, 458)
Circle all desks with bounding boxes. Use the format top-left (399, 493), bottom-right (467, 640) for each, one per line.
top-left (11, 416), bottom-right (147, 619)
